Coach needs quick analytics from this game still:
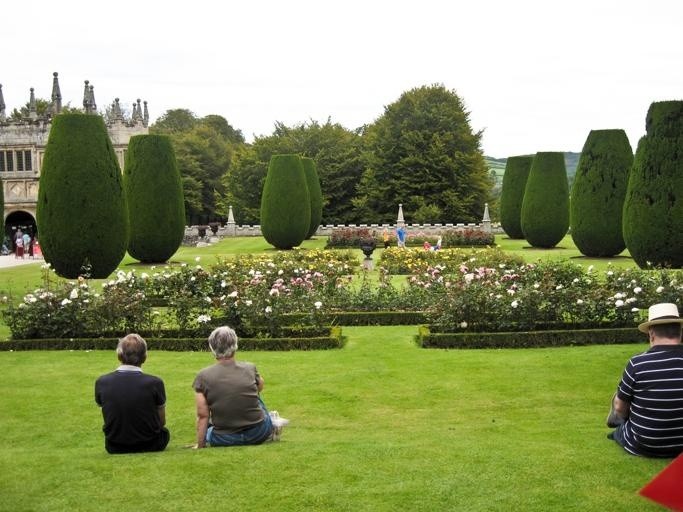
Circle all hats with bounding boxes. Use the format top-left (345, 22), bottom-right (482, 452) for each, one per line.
top-left (637, 302), bottom-right (683, 334)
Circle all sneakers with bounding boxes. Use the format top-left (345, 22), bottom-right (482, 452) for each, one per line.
top-left (606, 392), bottom-right (627, 429)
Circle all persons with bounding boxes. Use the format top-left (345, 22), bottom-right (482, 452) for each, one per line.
top-left (433, 236), bottom-right (442, 250)
top-left (94, 333), bottom-right (170, 454)
top-left (605, 303), bottom-right (682, 458)
top-left (0, 227), bottom-right (38, 261)
top-left (423, 237), bottom-right (434, 250)
top-left (382, 228), bottom-right (391, 249)
top-left (397, 223), bottom-right (407, 250)
top-left (182, 324), bottom-right (281, 449)
